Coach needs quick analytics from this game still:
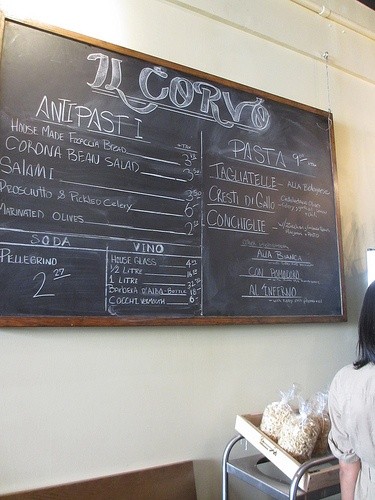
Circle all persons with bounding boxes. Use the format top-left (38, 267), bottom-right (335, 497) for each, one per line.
top-left (322, 280), bottom-right (375, 500)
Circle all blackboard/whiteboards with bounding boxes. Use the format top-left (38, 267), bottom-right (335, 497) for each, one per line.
top-left (0, 7), bottom-right (348, 328)
